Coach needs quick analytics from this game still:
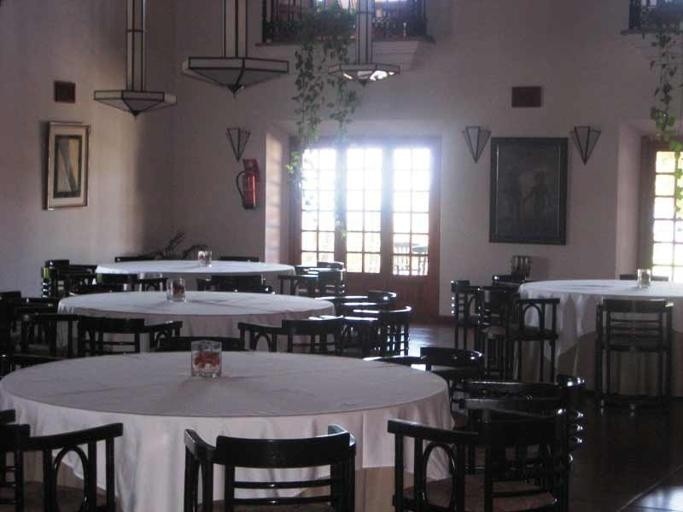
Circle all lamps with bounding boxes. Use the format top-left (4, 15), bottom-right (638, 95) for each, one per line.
top-left (570, 126), bottom-right (602, 166)
top-left (325, 0), bottom-right (402, 87)
top-left (181, 0), bottom-right (289, 97)
top-left (91, 0), bottom-right (178, 120)
top-left (223, 124), bottom-right (252, 160)
top-left (461, 123), bottom-right (492, 166)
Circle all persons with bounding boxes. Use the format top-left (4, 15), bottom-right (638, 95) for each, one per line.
top-left (522, 170), bottom-right (554, 229)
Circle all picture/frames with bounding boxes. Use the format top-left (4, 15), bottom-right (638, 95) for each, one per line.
top-left (41, 117), bottom-right (91, 213)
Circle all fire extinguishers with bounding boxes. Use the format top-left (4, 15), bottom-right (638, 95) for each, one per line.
top-left (235, 158), bottom-right (259, 210)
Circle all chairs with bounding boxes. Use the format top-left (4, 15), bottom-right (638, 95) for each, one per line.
top-left (1, 244), bottom-right (682, 512)
